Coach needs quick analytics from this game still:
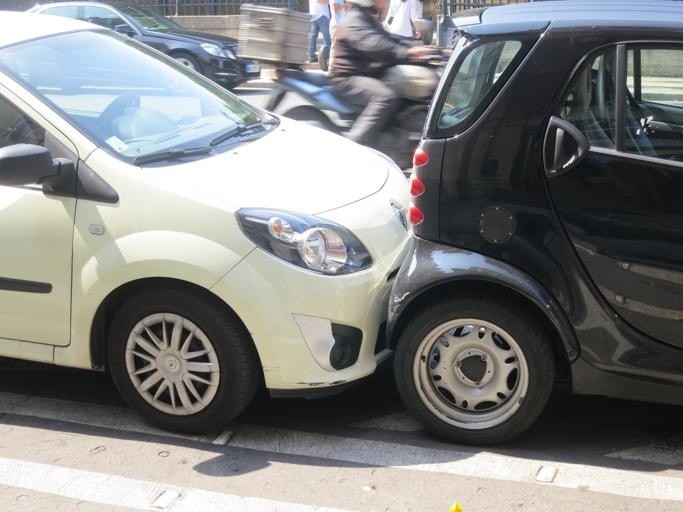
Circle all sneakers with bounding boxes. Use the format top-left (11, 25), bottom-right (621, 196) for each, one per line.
top-left (310, 56), bottom-right (318, 63)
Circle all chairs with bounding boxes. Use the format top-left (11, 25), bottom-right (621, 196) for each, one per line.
top-left (576, 58), bottom-right (657, 158)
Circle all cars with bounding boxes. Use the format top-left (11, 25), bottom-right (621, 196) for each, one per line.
top-left (384, 0), bottom-right (682, 449)
top-left (1, 1), bottom-right (410, 438)
top-left (23, 0), bottom-right (261, 90)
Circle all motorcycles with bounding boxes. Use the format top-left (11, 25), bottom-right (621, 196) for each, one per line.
top-left (265, 17), bottom-right (452, 180)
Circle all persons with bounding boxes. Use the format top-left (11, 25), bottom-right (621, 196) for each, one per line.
top-left (329, 0), bottom-right (352, 28)
top-left (382, 0), bottom-right (426, 40)
top-left (327, 0), bottom-right (441, 144)
top-left (308, 0), bottom-right (330, 62)
